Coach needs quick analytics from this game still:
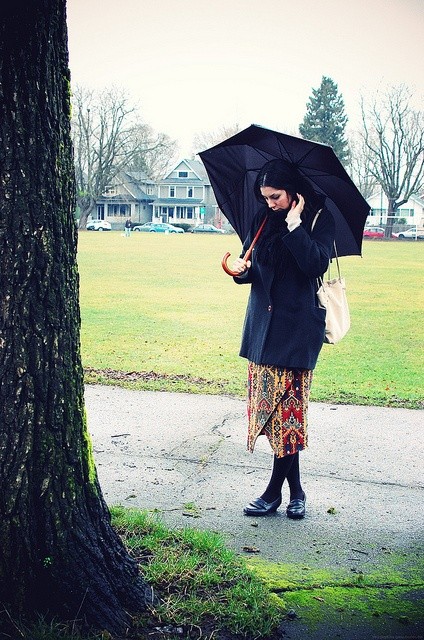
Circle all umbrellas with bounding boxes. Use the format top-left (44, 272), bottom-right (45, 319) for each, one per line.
top-left (195, 122), bottom-right (374, 277)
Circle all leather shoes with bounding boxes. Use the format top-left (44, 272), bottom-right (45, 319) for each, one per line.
top-left (244, 495), bottom-right (281, 515)
top-left (287, 491), bottom-right (306, 518)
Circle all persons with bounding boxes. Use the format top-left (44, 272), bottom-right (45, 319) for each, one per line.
top-left (124, 217), bottom-right (132, 238)
top-left (231, 159), bottom-right (337, 520)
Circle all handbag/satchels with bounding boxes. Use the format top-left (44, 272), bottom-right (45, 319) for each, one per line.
top-left (316, 277), bottom-right (351, 344)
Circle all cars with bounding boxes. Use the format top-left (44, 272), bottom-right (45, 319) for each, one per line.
top-left (150, 224), bottom-right (184, 233)
top-left (191, 224), bottom-right (224, 234)
top-left (363, 228), bottom-right (397, 240)
top-left (397, 228), bottom-right (424, 239)
top-left (86, 220), bottom-right (111, 231)
top-left (133, 222), bottom-right (152, 232)
